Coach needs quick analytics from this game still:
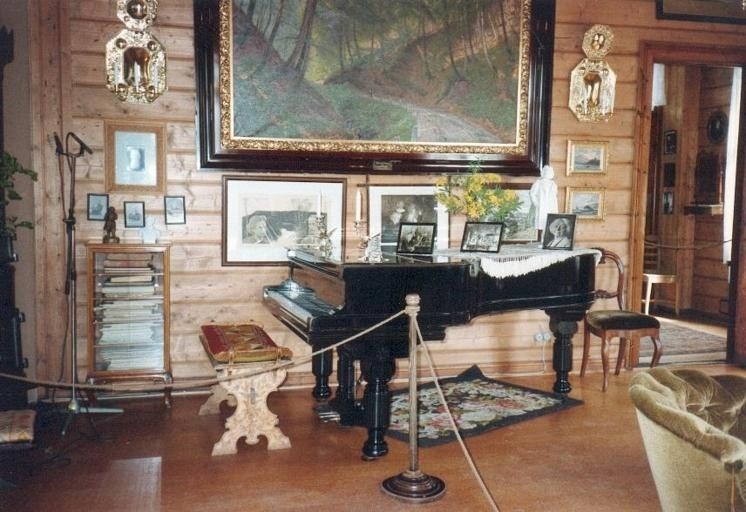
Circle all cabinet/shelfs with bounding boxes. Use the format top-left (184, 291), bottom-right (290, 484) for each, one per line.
top-left (84, 239), bottom-right (174, 408)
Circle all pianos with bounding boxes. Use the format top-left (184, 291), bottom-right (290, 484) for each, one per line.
top-left (263, 246), bottom-right (598, 460)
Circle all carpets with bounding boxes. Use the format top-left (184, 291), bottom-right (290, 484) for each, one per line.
top-left (330, 364), bottom-right (585, 448)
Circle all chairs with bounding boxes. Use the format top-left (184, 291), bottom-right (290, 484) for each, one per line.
top-left (579, 247), bottom-right (663, 392)
top-left (629, 367), bottom-right (746, 512)
top-left (642, 234), bottom-right (681, 316)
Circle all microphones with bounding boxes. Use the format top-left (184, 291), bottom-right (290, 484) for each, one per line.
top-left (70, 133), bottom-right (96, 155)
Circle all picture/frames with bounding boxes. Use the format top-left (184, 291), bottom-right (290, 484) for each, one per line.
top-left (662, 161), bottom-right (676, 185)
top-left (663, 130), bottom-right (677, 157)
top-left (656, 0), bottom-right (745, 25)
top-left (661, 190), bottom-right (673, 214)
top-left (704, 110), bottom-right (727, 142)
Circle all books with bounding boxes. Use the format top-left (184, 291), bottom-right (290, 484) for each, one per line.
top-left (94, 253), bottom-right (160, 367)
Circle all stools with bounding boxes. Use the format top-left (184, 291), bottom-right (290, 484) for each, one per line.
top-left (198, 323), bottom-right (294, 456)
top-left (0, 409), bottom-right (45, 476)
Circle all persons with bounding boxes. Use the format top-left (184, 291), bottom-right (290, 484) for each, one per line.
top-left (547, 217), bottom-right (571, 247)
top-left (103, 206), bottom-right (118, 238)
top-left (242, 214), bottom-right (269, 243)
top-left (528, 165), bottom-right (559, 230)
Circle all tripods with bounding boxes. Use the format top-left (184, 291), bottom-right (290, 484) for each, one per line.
top-left (45, 157), bottom-right (124, 435)
